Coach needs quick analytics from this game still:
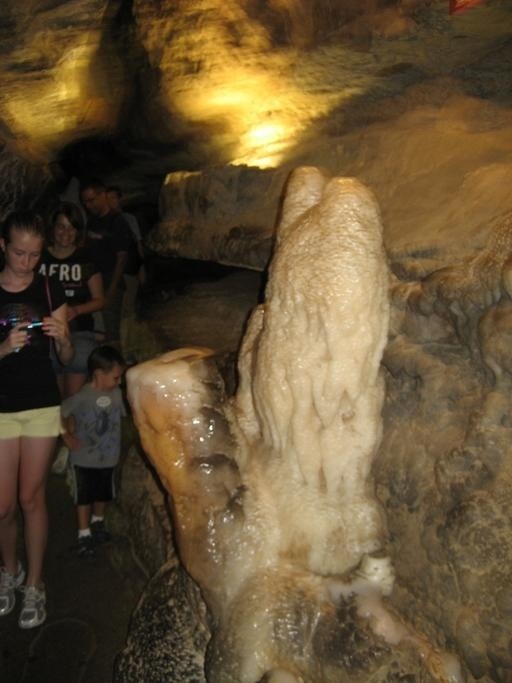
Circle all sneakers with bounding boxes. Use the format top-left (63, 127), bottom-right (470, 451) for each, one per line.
top-left (17, 582), bottom-right (49, 629)
top-left (1, 559), bottom-right (26, 617)
top-left (90, 519), bottom-right (108, 544)
top-left (77, 534), bottom-right (97, 560)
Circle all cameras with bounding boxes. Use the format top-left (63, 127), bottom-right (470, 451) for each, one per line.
top-left (0, 317), bottom-right (42, 331)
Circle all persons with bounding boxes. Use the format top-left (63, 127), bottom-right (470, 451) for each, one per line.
top-left (73, 183), bottom-right (144, 344)
top-left (33, 199), bottom-right (106, 371)
top-left (56, 347), bottom-right (134, 553)
top-left (0, 210), bottom-right (81, 637)
top-left (103, 186), bottom-right (147, 254)
